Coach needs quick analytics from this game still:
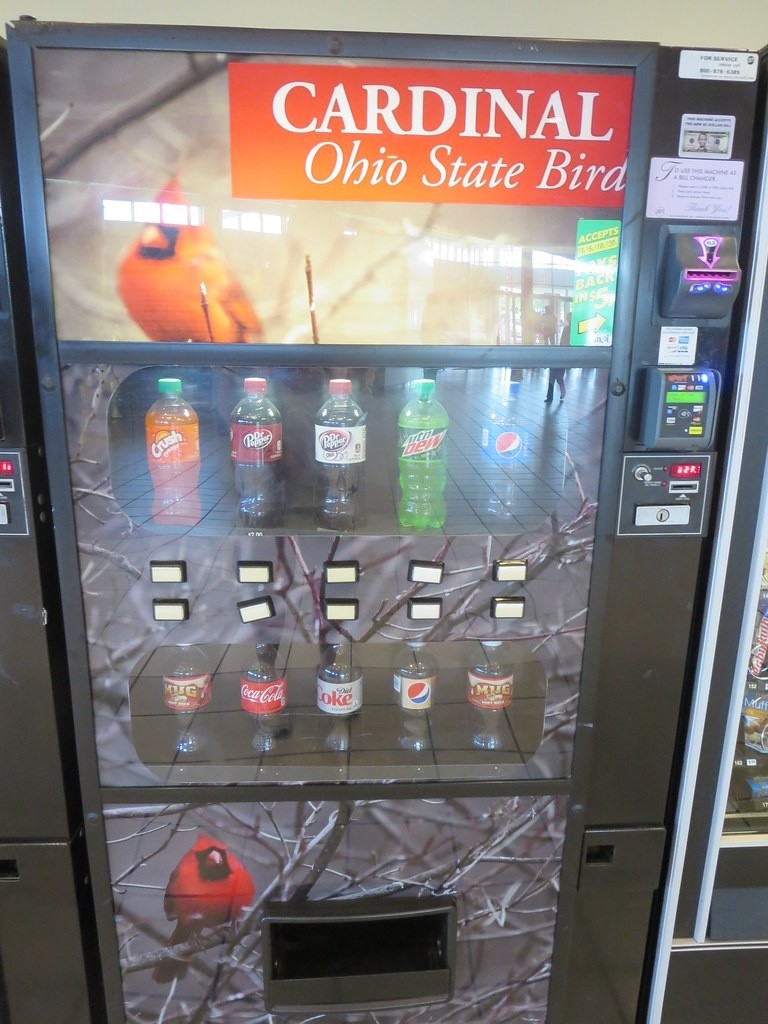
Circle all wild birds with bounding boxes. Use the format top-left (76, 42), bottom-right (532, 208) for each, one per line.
top-left (151, 833), bottom-right (256, 989)
top-left (111, 166), bottom-right (265, 346)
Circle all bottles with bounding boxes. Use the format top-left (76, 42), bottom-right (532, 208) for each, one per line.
top-left (313, 379), bottom-right (366, 531)
top-left (398, 379), bottom-right (449, 529)
top-left (240, 645), bottom-right (289, 752)
top-left (480, 381), bottom-right (528, 519)
top-left (230, 378), bottom-right (282, 528)
top-left (162, 644), bottom-right (211, 753)
top-left (468, 641), bottom-right (513, 748)
top-left (144, 379), bottom-right (202, 526)
top-left (315, 644), bottom-right (362, 750)
top-left (392, 641), bottom-right (439, 751)
